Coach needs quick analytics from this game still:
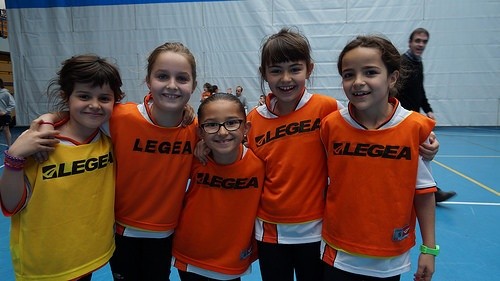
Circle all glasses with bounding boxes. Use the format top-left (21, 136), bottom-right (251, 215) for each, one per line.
top-left (201, 119), bottom-right (247, 134)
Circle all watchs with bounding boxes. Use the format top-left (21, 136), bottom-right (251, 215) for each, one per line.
top-left (421, 245), bottom-right (439, 256)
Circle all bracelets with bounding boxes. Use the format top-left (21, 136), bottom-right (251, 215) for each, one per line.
top-left (4, 151), bottom-right (25, 170)
top-left (39, 121), bottom-right (54, 126)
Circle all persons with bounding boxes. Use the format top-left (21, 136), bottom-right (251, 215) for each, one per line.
top-left (31, 42), bottom-right (198, 281)
top-left (173, 93), bottom-right (264, 281)
top-left (259, 95), bottom-right (266, 104)
top-left (396, 29), bottom-right (455, 202)
top-left (194, 32), bottom-right (439, 281)
top-left (200, 83), bottom-right (248, 108)
top-left (320, 37), bottom-right (435, 281)
top-left (0, 54), bottom-right (126, 281)
top-left (0, 76), bottom-right (15, 148)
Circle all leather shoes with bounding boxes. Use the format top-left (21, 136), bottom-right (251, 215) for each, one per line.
top-left (435, 186), bottom-right (456, 205)
